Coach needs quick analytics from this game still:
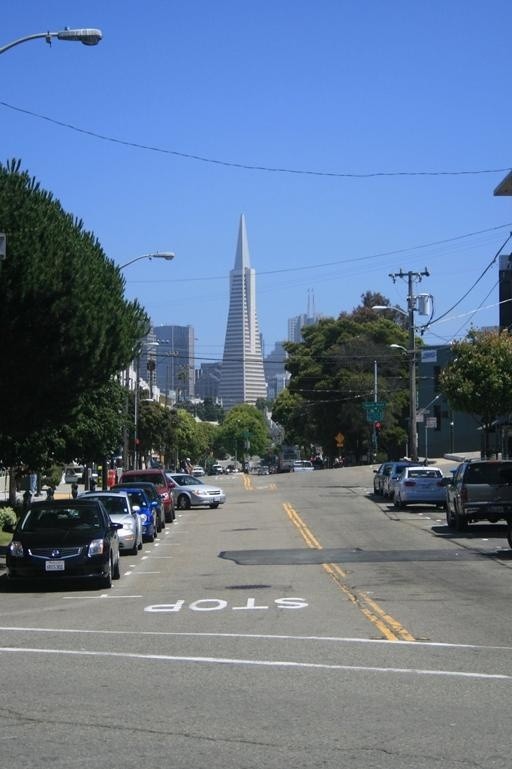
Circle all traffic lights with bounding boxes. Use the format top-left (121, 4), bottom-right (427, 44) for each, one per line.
top-left (376, 421), bottom-right (381, 431)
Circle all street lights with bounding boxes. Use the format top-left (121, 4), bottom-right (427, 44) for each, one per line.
top-left (371, 304), bottom-right (418, 463)
top-left (133, 339), bottom-right (161, 472)
top-left (116, 251), bottom-right (176, 271)
top-left (1, 26), bottom-right (103, 56)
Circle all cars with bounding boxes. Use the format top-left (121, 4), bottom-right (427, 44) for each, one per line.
top-left (372, 462), bottom-right (396, 494)
top-left (393, 467), bottom-right (446, 509)
top-left (187, 459), bottom-right (318, 477)
top-left (7, 470), bottom-right (176, 587)
top-left (383, 463), bottom-right (423, 497)
top-left (165, 472), bottom-right (226, 510)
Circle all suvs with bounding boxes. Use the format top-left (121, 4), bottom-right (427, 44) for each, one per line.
top-left (441, 459), bottom-right (511, 529)
top-left (65, 466), bottom-right (99, 485)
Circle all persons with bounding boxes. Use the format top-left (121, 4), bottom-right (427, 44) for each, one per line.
top-left (178, 451), bottom-right (345, 477)
